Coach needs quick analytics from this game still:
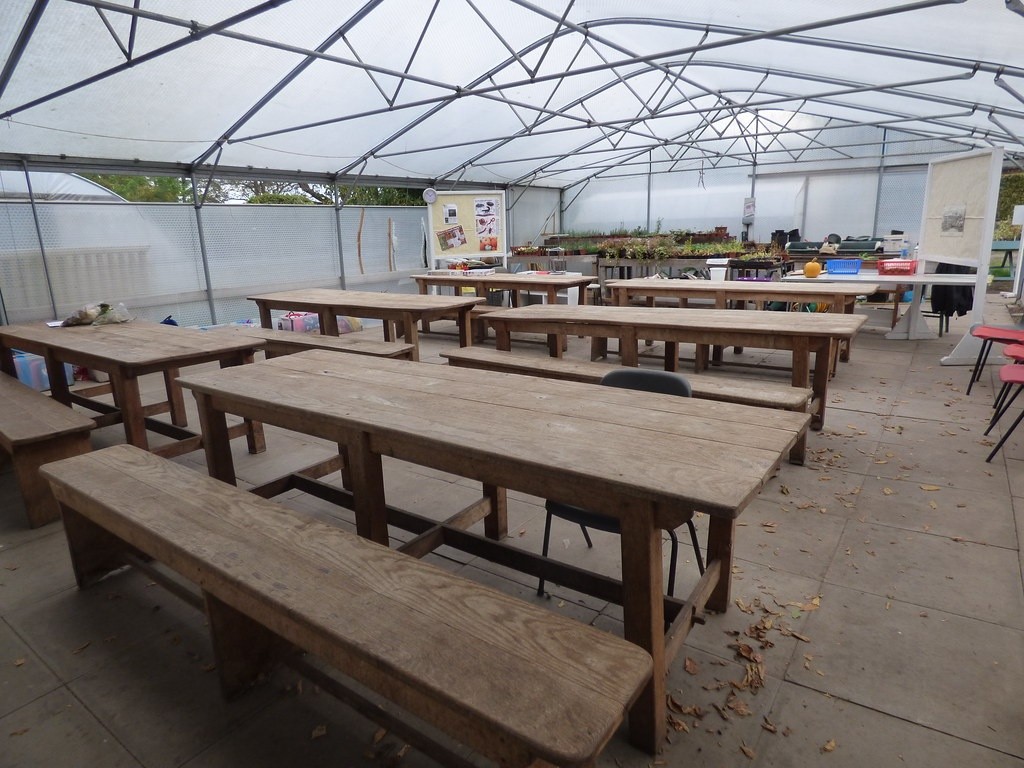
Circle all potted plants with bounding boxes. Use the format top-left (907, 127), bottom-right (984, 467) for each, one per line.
top-left (578, 242), bottom-right (589, 255)
top-left (596, 238), bottom-right (747, 260)
top-left (557, 242), bottom-right (575, 257)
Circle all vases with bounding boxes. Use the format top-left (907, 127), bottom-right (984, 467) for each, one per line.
top-left (515, 252), bottom-right (540, 257)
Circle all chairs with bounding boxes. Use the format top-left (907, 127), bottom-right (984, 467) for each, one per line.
top-left (537, 369), bottom-right (706, 597)
top-left (966, 323), bottom-right (1024, 397)
top-left (984, 364), bottom-right (1024, 463)
top-left (990, 344), bottom-right (1024, 423)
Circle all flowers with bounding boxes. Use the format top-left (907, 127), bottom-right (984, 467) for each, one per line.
top-left (514, 246), bottom-right (539, 252)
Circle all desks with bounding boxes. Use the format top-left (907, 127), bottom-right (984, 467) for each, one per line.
top-left (173, 346), bottom-right (812, 756)
top-left (781, 270), bottom-right (994, 346)
top-left (606, 275), bottom-right (880, 363)
top-left (1, 315), bottom-right (268, 463)
top-left (245, 288), bottom-right (487, 363)
top-left (477, 303), bottom-right (869, 431)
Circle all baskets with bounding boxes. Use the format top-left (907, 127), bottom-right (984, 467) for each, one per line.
top-left (877, 258), bottom-right (917, 276)
top-left (826, 260), bottom-right (864, 274)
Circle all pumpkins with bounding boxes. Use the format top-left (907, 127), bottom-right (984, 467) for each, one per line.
top-left (803, 258), bottom-right (821, 278)
top-left (479, 236), bottom-right (497, 250)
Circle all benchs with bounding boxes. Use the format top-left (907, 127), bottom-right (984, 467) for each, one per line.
top-left (990, 240), bottom-right (1020, 280)
top-left (411, 272), bottom-right (598, 351)
top-left (0, 304), bottom-right (847, 764)
